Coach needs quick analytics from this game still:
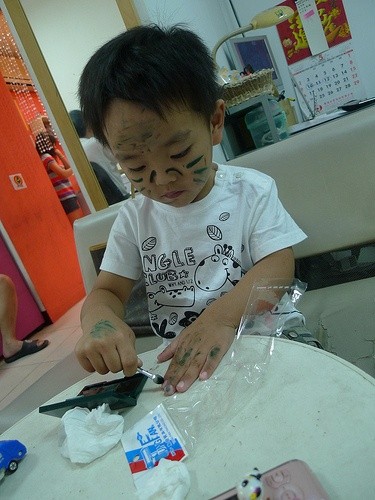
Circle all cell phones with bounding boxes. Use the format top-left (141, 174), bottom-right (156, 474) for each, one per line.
top-left (208, 459), bottom-right (329, 500)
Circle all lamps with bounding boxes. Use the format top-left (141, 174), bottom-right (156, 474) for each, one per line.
top-left (211, 5), bottom-right (295, 64)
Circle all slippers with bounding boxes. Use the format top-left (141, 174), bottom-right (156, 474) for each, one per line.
top-left (5, 339), bottom-right (48, 363)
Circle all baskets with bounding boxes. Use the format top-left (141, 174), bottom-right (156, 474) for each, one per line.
top-left (220, 67), bottom-right (274, 108)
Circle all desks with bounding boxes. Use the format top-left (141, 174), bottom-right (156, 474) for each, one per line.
top-left (0, 333), bottom-right (375, 500)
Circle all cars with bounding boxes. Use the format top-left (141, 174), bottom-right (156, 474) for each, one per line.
top-left (0, 439), bottom-right (26, 485)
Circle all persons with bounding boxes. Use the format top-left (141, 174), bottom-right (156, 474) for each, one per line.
top-left (69, 109), bottom-right (131, 206)
top-left (74, 19), bottom-right (324, 396)
top-left (0, 274), bottom-right (49, 364)
top-left (35, 132), bottom-right (82, 229)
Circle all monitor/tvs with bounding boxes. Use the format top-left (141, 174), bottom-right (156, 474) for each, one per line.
top-left (222, 35), bottom-right (282, 87)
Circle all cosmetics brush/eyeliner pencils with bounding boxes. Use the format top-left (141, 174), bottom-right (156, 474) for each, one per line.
top-left (137, 367), bottom-right (165, 384)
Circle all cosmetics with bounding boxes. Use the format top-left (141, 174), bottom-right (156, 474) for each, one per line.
top-left (39, 373), bottom-right (149, 418)
